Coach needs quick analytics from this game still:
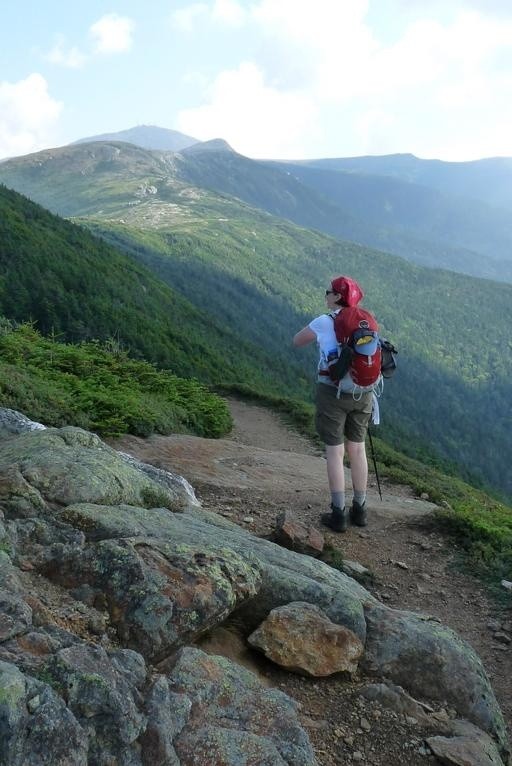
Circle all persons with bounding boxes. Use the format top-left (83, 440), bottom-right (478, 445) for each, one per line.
top-left (293, 276), bottom-right (382, 533)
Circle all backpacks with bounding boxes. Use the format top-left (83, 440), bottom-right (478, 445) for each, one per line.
top-left (318, 306), bottom-right (382, 386)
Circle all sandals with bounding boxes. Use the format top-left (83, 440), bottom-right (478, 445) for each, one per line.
top-left (381, 340), bottom-right (398, 378)
top-left (329, 343), bottom-right (355, 381)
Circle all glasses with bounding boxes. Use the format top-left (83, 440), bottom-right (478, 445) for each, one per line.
top-left (326, 290), bottom-right (335, 295)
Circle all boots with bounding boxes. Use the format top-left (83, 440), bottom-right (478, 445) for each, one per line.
top-left (349, 499), bottom-right (368, 526)
top-left (321, 502), bottom-right (347, 533)
